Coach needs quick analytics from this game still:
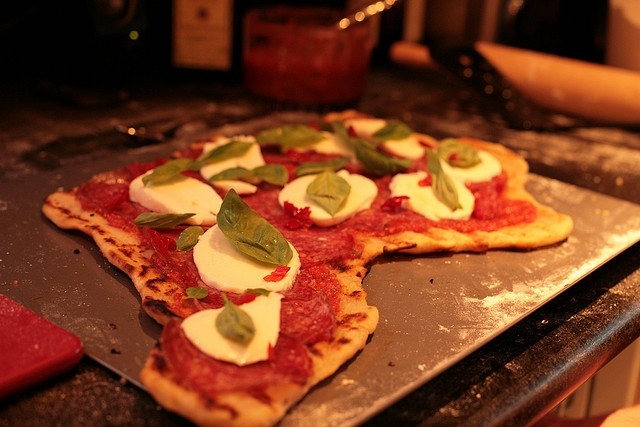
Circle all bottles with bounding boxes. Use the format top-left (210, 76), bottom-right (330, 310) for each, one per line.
top-left (172, 0), bottom-right (234, 81)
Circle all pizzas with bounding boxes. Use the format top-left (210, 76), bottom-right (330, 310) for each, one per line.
top-left (42, 106), bottom-right (575, 426)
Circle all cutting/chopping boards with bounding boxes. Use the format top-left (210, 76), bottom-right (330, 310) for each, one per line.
top-left (0, 114), bottom-right (640, 427)
